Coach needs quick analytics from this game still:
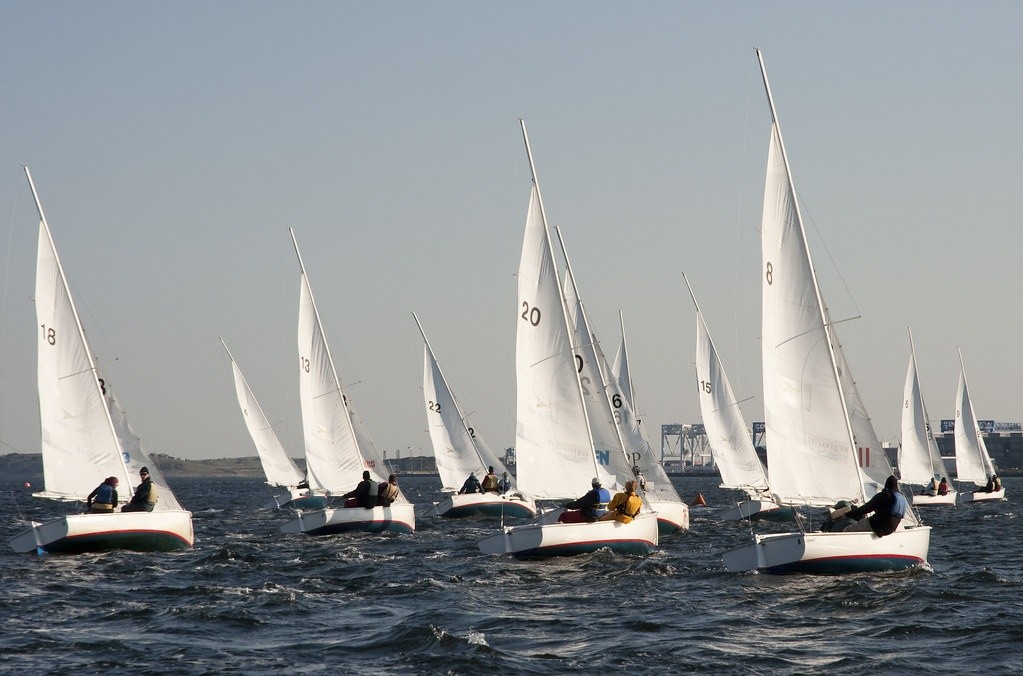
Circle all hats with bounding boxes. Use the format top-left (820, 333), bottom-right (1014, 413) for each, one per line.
top-left (363, 471), bottom-right (370, 477)
top-left (992, 474), bottom-right (998, 479)
top-left (104, 477), bottom-right (120, 485)
top-left (140, 466), bottom-right (150, 474)
top-left (593, 477), bottom-right (601, 485)
top-left (885, 475), bottom-right (898, 488)
top-left (502, 471), bottom-right (508, 478)
top-left (470, 473), bottom-right (475, 476)
top-left (488, 465), bottom-right (494, 472)
top-left (388, 474), bottom-right (396, 480)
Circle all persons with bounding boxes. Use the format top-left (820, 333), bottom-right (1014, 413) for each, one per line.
top-left (375, 475), bottom-right (398, 507)
top-left (86, 477), bottom-right (118, 513)
top-left (843, 475), bottom-right (906, 538)
top-left (498, 472), bottom-right (511, 491)
top-left (342, 471), bottom-right (378, 509)
top-left (458, 472), bottom-right (485, 494)
top-left (482, 466), bottom-right (500, 492)
top-left (121, 467), bottom-right (159, 512)
top-left (599, 480), bottom-right (642, 524)
top-left (979, 475), bottom-right (1001, 492)
top-left (632, 466), bottom-right (644, 489)
top-left (922, 478), bottom-right (939, 496)
top-left (558, 478), bottom-right (610, 523)
top-left (938, 477), bottom-right (948, 496)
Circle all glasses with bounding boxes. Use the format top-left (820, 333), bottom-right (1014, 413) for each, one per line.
top-left (140, 473), bottom-right (147, 476)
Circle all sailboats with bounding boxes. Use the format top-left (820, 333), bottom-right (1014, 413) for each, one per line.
top-left (946, 347), bottom-right (1005, 502)
top-left (553, 227), bottom-right (698, 536)
top-left (4, 161), bottom-right (194, 563)
top-left (892, 324), bottom-right (960, 508)
top-left (218, 224), bottom-right (422, 536)
top-left (472, 116), bottom-right (662, 561)
top-left (404, 311), bottom-right (540, 519)
top-left (679, 270), bottom-right (789, 525)
top-left (700, 48), bottom-right (935, 572)
